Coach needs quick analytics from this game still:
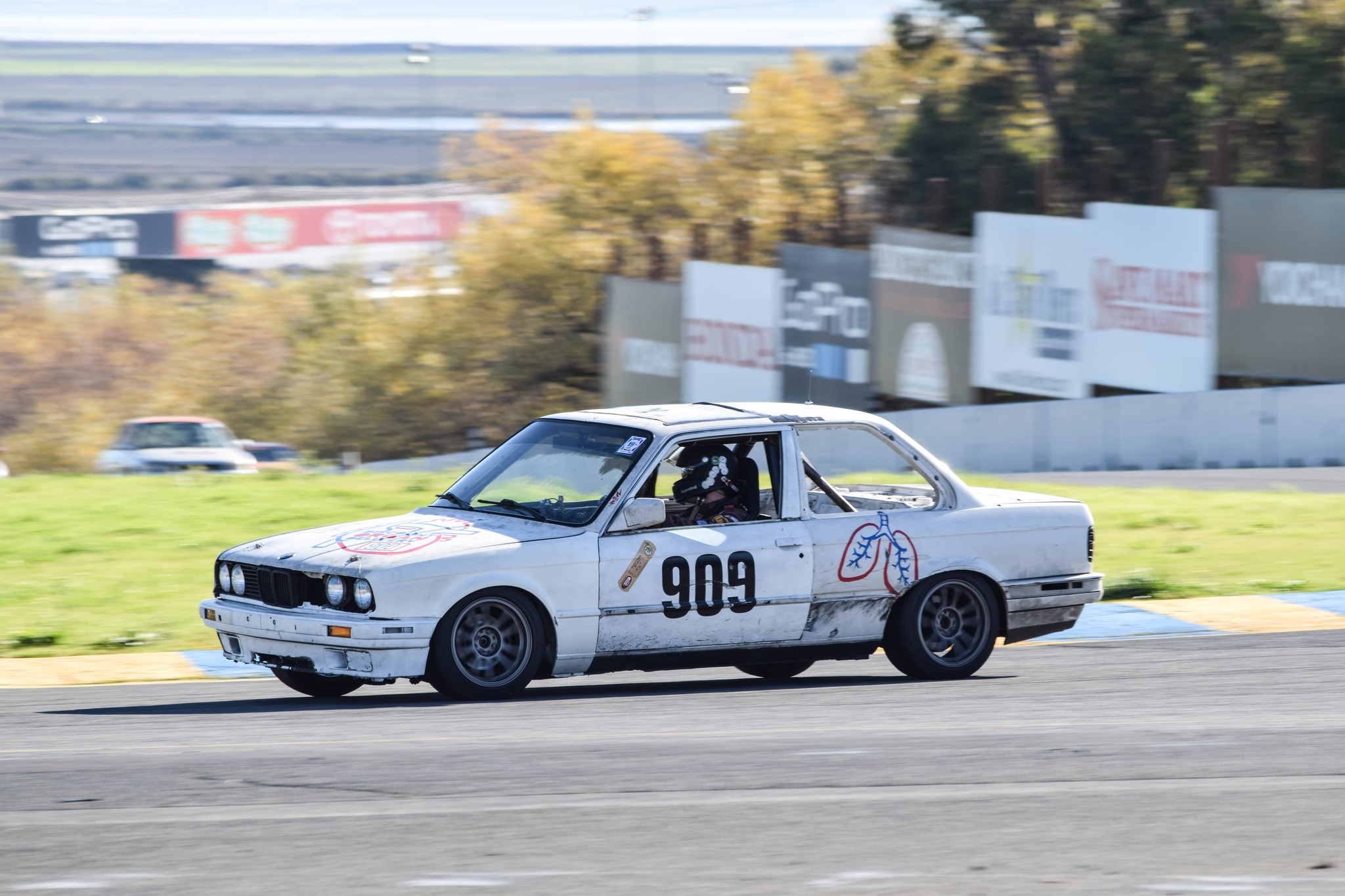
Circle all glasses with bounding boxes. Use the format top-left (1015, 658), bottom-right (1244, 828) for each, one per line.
top-left (682, 469), bottom-right (694, 478)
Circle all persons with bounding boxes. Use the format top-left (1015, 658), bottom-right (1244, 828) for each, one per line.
top-left (651, 440), bottom-right (746, 527)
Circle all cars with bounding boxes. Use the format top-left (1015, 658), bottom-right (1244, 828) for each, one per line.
top-left (98, 415), bottom-right (257, 474)
top-left (236, 436), bottom-right (311, 474)
top-left (197, 397), bottom-right (1104, 704)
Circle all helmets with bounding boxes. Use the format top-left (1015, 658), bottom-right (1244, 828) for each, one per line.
top-left (668, 441), bottom-right (740, 502)
